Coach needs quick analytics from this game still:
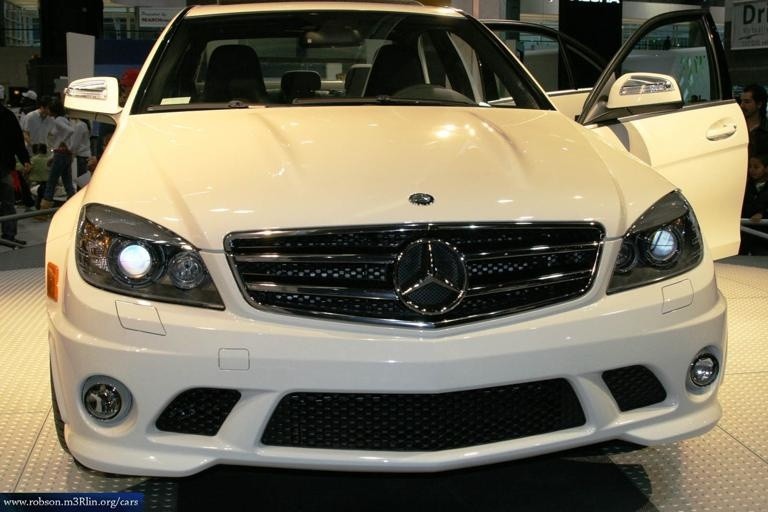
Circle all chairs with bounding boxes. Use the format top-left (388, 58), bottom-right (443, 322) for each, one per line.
top-left (279, 70), bottom-right (321, 103)
top-left (344, 65), bottom-right (372, 96)
top-left (209, 44), bottom-right (271, 103)
top-left (357, 44), bottom-right (422, 100)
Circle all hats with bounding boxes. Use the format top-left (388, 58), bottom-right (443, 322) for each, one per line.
top-left (22, 90), bottom-right (37, 100)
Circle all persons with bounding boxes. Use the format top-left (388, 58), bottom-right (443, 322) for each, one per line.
top-left (661, 35), bottom-right (673, 51)
top-left (747, 150), bottom-right (767, 226)
top-left (1, 87), bottom-right (102, 253)
top-left (690, 94), bottom-right (699, 103)
top-left (738, 81), bottom-right (767, 158)
top-left (111, 64), bottom-right (140, 105)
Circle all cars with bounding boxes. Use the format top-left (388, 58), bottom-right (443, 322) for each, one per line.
top-left (44, 0), bottom-right (750, 481)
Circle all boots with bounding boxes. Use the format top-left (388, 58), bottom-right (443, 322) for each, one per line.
top-left (33, 199), bottom-right (54, 221)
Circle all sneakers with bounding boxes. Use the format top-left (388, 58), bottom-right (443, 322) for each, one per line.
top-left (0, 234), bottom-right (27, 248)
